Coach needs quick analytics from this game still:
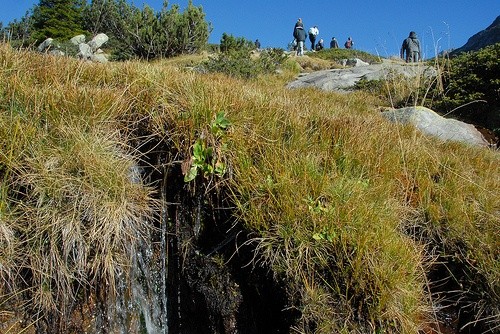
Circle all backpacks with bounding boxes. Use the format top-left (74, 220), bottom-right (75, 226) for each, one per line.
top-left (345, 40), bottom-right (352, 48)
top-left (308, 28), bottom-right (316, 35)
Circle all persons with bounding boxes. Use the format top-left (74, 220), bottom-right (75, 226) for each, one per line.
top-left (318, 39), bottom-right (324, 50)
top-left (293, 18), bottom-right (307, 55)
top-left (255, 39), bottom-right (260, 48)
top-left (345, 37), bottom-right (353, 48)
top-left (400, 32), bottom-right (421, 62)
top-left (330, 37), bottom-right (338, 48)
top-left (309, 26), bottom-right (319, 50)
